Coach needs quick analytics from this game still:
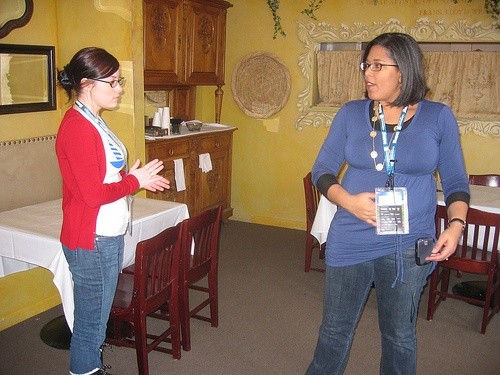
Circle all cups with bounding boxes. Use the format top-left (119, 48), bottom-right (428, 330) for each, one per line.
top-left (152, 107), bottom-right (171, 135)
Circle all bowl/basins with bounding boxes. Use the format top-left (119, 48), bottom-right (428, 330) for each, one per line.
top-left (186, 122), bottom-right (202, 131)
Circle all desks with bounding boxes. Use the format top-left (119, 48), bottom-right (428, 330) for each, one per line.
top-left (310, 180), bottom-right (500, 296)
top-left (0, 192), bottom-right (190, 350)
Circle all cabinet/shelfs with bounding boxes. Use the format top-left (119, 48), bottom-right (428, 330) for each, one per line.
top-left (145, 121), bottom-right (237, 223)
top-left (142, 0), bottom-right (233, 90)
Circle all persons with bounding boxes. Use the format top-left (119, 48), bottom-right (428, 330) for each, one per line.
top-left (55, 47), bottom-right (170, 375)
top-left (305, 32), bottom-right (470, 375)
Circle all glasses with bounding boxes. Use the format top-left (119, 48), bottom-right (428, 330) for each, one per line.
top-left (85, 77), bottom-right (125, 88)
top-left (359, 62), bottom-right (399, 71)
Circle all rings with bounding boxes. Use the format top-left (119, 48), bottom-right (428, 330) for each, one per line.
top-left (446, 257), bottom-right (448, 260)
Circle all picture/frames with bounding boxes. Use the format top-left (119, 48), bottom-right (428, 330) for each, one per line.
top-left (0, 43), bottom-right (56, 116)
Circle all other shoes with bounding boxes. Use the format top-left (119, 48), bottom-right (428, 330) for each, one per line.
top-left (91, 364), bottom-right (114, 375)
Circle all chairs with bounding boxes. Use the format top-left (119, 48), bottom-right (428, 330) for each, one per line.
top-left (304, 170), bottom-right (500, 375)
top-left (101, 203), bottom-right (224, 375)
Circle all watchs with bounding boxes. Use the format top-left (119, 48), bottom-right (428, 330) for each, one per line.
top-left (447, 219), bottom-right (466, 230)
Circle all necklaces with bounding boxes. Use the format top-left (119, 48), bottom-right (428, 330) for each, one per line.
top-left (370, 104), bottom-right (404, 171)
